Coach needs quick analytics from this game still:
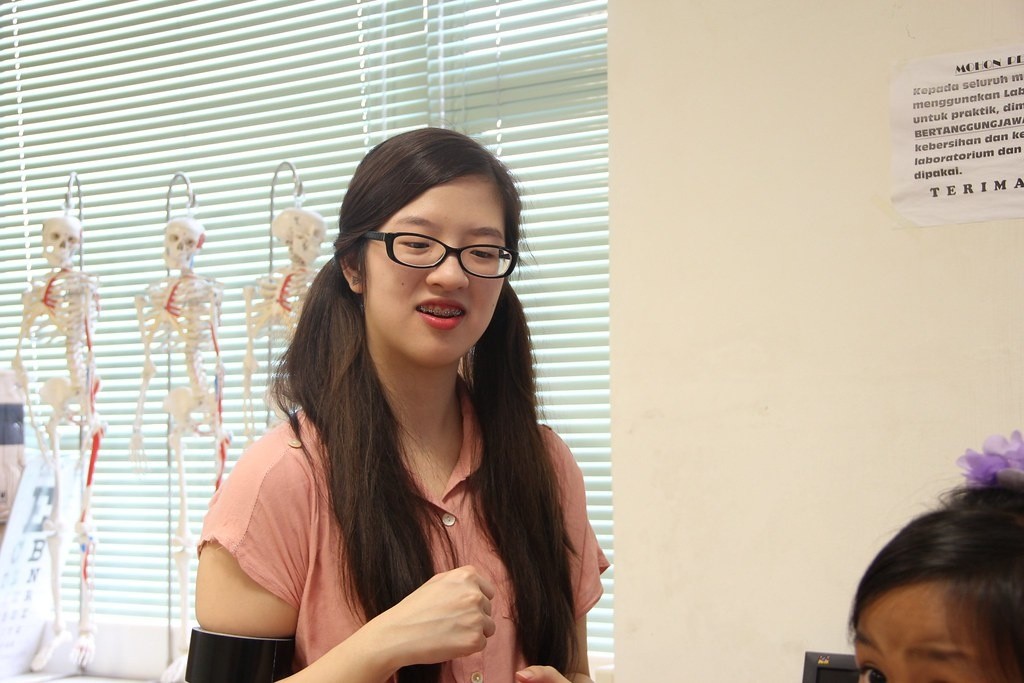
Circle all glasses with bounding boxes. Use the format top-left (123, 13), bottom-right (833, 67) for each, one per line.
top-left (364, 231), bottom-right (519, 279)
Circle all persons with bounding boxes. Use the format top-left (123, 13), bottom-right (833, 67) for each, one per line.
top-left (853, 432), bottom-right (1023, 682)
top-left (188, 127), bottom-right (611, 683)
